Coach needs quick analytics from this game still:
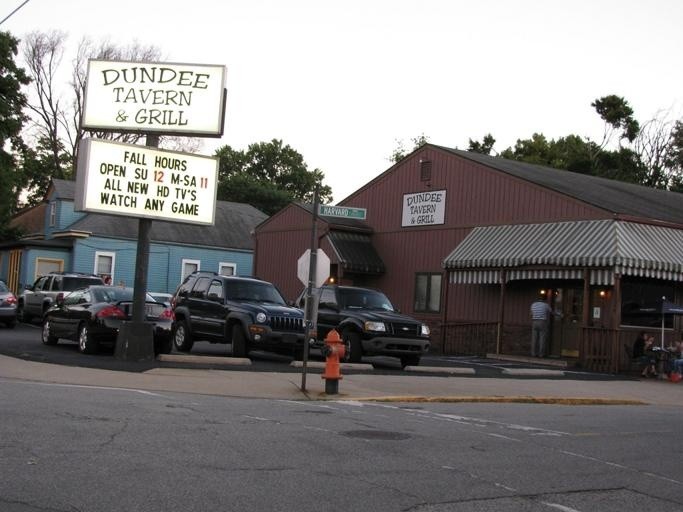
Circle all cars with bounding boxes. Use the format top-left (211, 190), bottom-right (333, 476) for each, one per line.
top-left (0, 280), bottom-right (19, 331)
top-left (41, 284), bottom-right (175, 356)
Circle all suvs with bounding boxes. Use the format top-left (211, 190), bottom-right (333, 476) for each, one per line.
top-left (295, 283), bottom-right (432, 367)
top-left (169, 268), bottom-right (316, 360)
top-left (16, 270), bottom-right (107, 324)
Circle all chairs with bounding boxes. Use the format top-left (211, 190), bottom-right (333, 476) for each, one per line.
top-left (623, 343), bottom-right (683, 382)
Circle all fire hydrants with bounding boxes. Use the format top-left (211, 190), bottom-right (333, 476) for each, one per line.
top-left (319, 329), bottom-right (349, 394)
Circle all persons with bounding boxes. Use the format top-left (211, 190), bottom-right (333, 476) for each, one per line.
top-left (665, 337), bottom-right (682, 373)
top-left (527, 294), bottom-right (560, 358)
top-left (632, 330), bottom-right (659, 378)
top-left (103, 276), bottom-right (113, 286)
